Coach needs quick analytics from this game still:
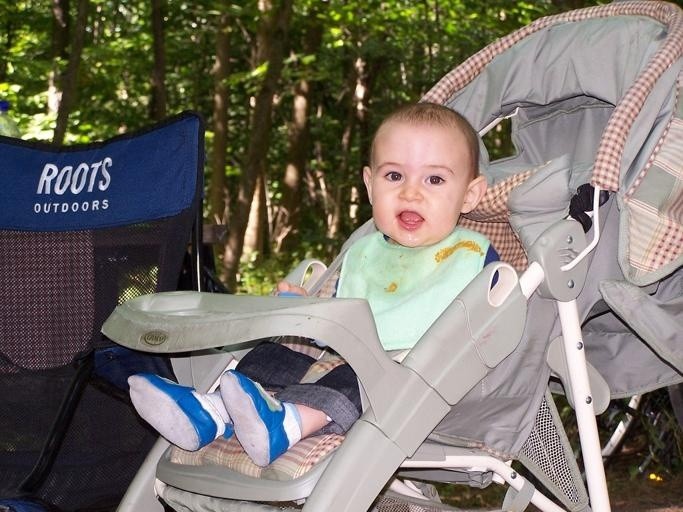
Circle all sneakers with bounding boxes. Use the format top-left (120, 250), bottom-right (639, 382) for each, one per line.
top-left (220, 369), bottom-right (289, 468)
top-left (127, 373), bottom-right (218, 452)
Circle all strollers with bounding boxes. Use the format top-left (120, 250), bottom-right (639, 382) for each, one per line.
top-left (0, 0), bottom-right (683, 512)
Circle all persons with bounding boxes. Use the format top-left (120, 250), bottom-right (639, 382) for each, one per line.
top-left (126, 99), bottom-right (502, 469)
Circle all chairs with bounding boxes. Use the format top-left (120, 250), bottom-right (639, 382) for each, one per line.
top-left (1, 109), bottom-right (228, 511)
